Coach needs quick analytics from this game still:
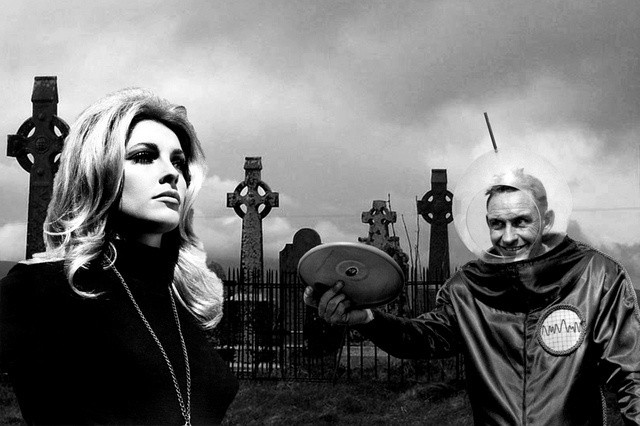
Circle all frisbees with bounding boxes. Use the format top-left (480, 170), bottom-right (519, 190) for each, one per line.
top-left (297, 241), bottom-right (406, 309)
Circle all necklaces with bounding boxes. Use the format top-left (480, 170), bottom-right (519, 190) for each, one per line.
top-left (100, 249), bottom-right (192, 426)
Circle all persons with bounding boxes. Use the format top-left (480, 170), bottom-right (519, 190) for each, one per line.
top-left (1, 85), bottom-right (244, 426)
top-left (302, 167), bottom-right (639, 426)
